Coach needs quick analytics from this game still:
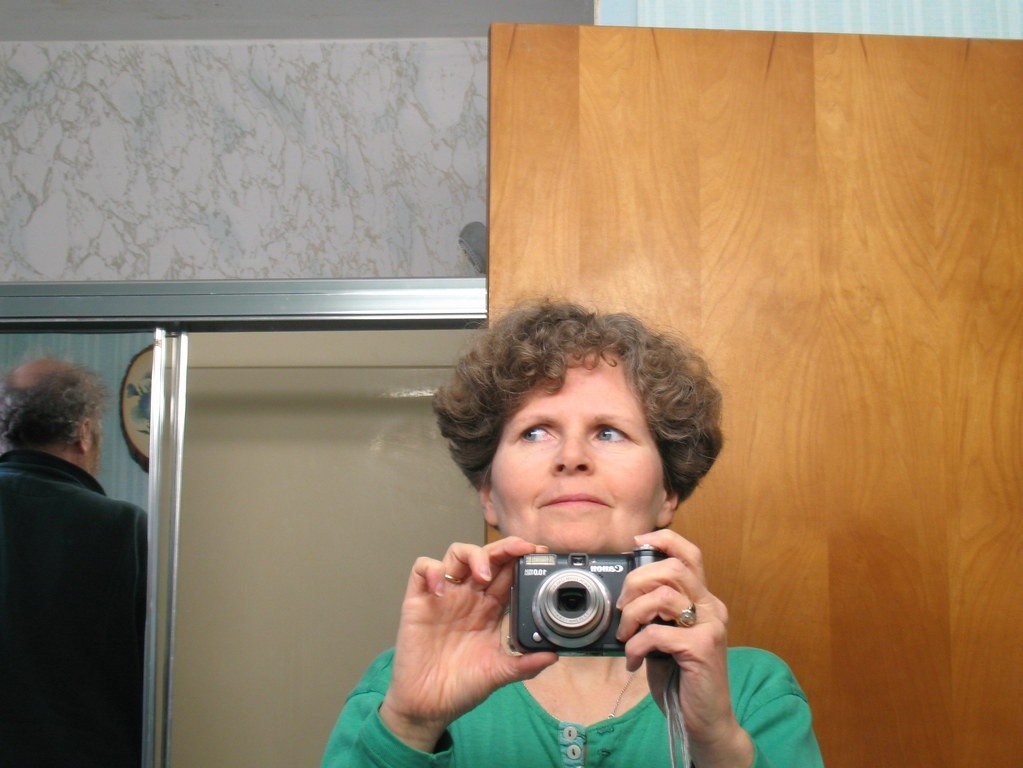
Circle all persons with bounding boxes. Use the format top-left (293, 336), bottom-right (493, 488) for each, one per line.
top-left (0, 359), bottom-right (148, 768)
top-left (319, 294), bottom-right (825, 768)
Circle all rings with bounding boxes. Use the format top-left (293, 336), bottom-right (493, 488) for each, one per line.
top-left (675, 601), bottom-right (697, 628)
top-left (444, 573), bottom-right (463, 584)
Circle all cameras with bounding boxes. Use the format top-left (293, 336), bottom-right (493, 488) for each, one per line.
top-left (507, 544), bottom-right (679, 661)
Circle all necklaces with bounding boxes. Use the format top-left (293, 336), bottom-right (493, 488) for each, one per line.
top-left (608, 672), bottom-right (635, 719)
